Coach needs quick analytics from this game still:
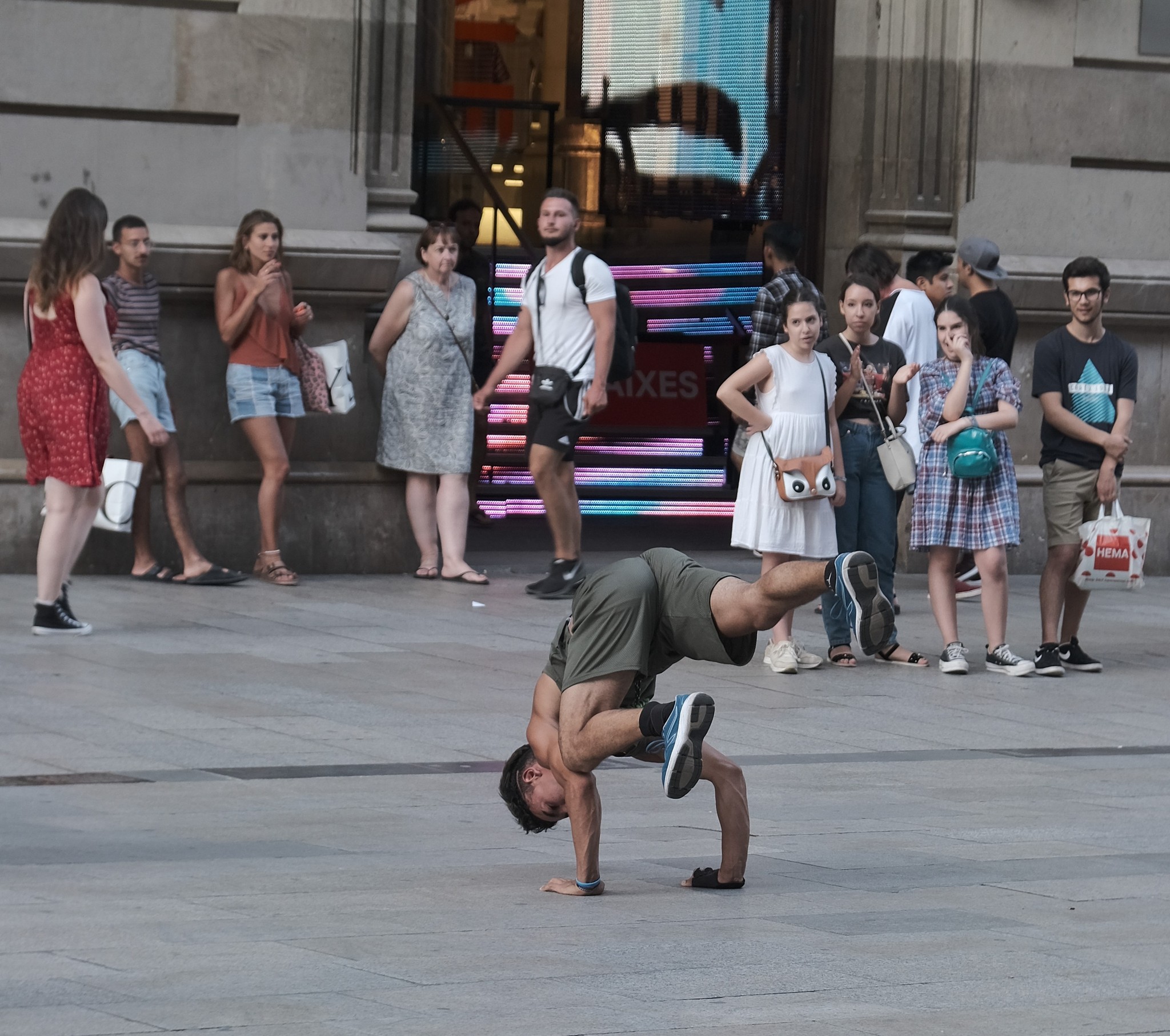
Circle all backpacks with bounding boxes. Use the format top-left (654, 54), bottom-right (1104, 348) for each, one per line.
top-left (931, 356), bottom-right (999, 478)
top-left (521, 247), bottom-right (640, 385)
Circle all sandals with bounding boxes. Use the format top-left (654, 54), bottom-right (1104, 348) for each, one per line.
top-left (874, 643), bottom-right (929, 667)
top-left (253, 559), bottom-right (299, 586)
top-left (827, 641), bottom-right (857, 667)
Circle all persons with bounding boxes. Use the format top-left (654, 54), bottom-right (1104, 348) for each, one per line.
top-left (100, 216), bottom-right (249, 585)
top-left (715, 215), bottom-right (1139, 675)
top-left (15, 190), bottom-right (173, 638)
top-left (499, 539), bottom-right (896, 896)
top-left (476, 189), bottom-right (618, 602)
top-left (369, 199), bottom-right (492, 581)
top-left (214, 209), bottom-right (313, 584)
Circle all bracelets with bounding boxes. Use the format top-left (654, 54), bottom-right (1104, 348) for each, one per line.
top-left (575, 877), bottom-right (601, 892)
top-left (967, 415), bottom-right (980, 428)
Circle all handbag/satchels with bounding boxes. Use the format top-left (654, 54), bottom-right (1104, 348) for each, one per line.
top-left (1065, 497), bottom-right (1151, 589)
top-left (876, 413), bottom-right (917, 490)
top-left (772, 446), bottom-right (837, 502)
top-left (529, 365), bottom-right (574, 406)
top-left (309, 339), bottom-right (356, 415)
top-left (40, 454), bottom-right (143, 534)
top-left (293, 335), bottom-right (332, 414)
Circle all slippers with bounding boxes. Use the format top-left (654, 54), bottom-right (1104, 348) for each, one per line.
top-left (186, 561), bottom-right (249, 584)
top-left (131, 560), bottom-right (185, 584)
top-left (413, 565), bottom-right (439, 579)
top-left (441, 570), bottom-right (489, 585)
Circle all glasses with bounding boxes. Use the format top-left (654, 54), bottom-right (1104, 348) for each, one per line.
top-left (537, 276), bottom-right (546, 305)
top-left (1064, 285), bottom-right (1106, 303)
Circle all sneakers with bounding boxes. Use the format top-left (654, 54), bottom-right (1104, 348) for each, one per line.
top-left (645, 692), bottom-right (715, 800)
top-left (985, 643), bottom-right (1036, 676)
top-left (525, 557), bottom-right (587, 599)
top-left (33, 583), bottom-right (94, 635)
top-left (926, 578), bottom-right (983, 598)
top-left (1032, 644), bottom-right (1065, 676)
top-left (768, 640), bottom-right (801, 674)
top-left (762, 635), bottom-right (824, 668)
top-left (827, 549), bottom-right (895, 658)
top-left (939, 641), bottom-right (969, 675)
top-left (954, 561), bottom-right (983, 585)
top-left (1058, 634), bottom-right (1104, 671)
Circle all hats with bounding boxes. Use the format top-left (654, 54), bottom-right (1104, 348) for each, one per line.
top-left (959, 236), bottom-right (1008, 279)
